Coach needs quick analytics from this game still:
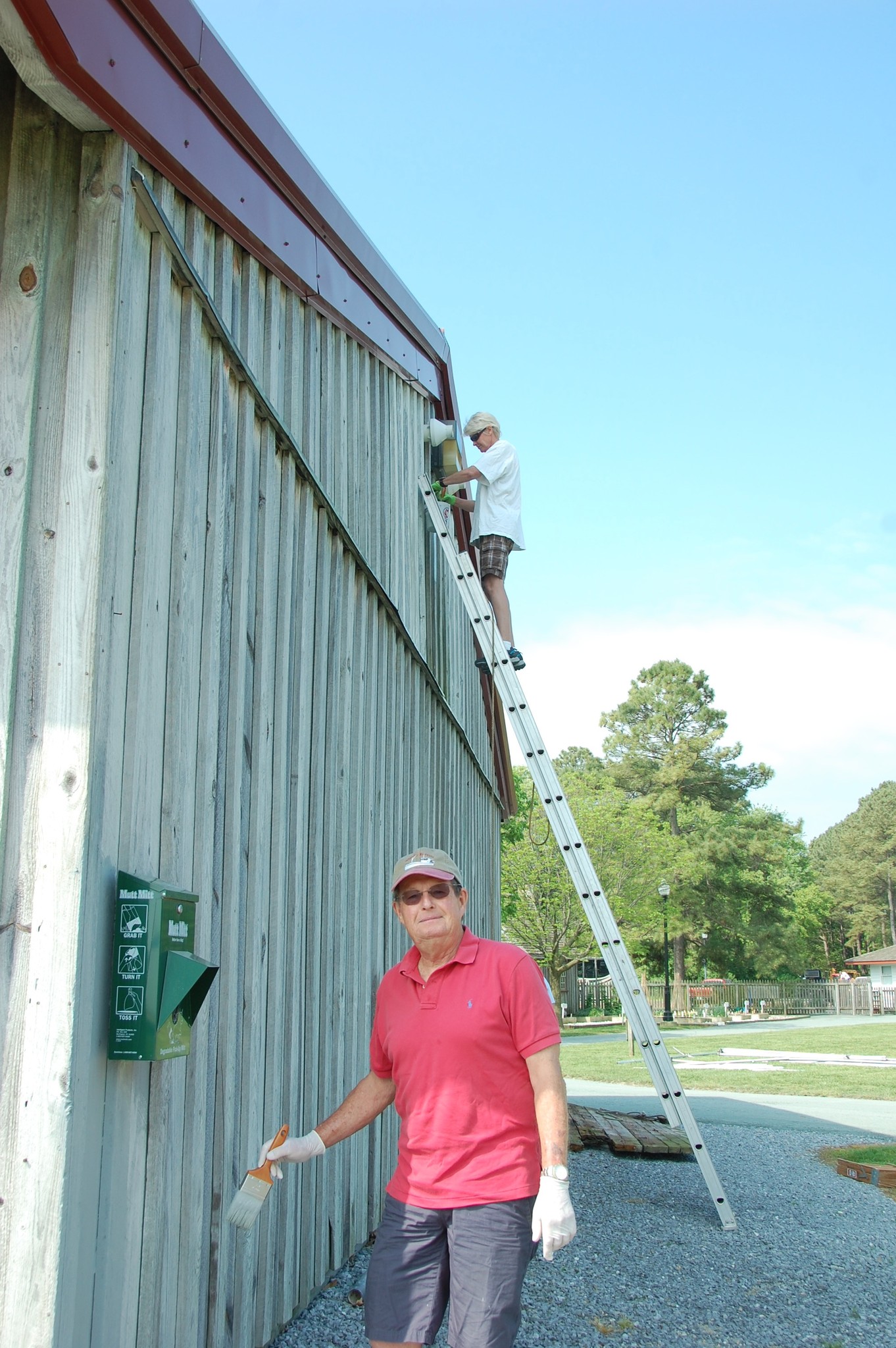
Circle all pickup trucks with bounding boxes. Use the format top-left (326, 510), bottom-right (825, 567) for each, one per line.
top-left (689, 979), bottom-right (733, 1005)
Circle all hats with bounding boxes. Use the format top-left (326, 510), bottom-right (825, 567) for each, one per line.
top-left (391, 847), bottom-right (466, 891)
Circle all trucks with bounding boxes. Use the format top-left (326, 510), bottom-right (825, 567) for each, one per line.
top-left (829, 968), bottom-right (861, 984)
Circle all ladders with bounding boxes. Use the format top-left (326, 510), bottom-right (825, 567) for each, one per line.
top-left (418, 474), bottom-right (734, 1231)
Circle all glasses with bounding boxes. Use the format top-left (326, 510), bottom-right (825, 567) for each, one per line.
top-left (397, 883), bottom-right (462, 905)
top-left (471, 427), bottom-right (493, 442)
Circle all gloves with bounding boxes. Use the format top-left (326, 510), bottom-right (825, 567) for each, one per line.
top-left (532, 1174), bottom-right (578, 1261)
top-left (437, 491), bottom-right (455, 505)
top-left (431, 481), bottom-right (442, 495)
top-left (259, 1129), bottom-right (326, 1180)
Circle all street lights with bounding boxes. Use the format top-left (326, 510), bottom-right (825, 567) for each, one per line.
top-left (658, 877), bottom-right (673, 1021)
top-left (702, 931), bottom-right (708, 980)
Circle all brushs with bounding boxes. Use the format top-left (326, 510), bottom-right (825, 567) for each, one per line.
top-left (223, 1122), bottom-right (291, 1228)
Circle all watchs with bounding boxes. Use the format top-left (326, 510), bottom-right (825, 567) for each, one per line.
top-left (438, 477), bottom-right (448, 487)
top-left (541, 1164), bottom-right (569, 1181)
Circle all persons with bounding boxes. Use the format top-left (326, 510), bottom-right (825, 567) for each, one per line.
top-left (258, 848), bottom-right (578, 1348)
top-left (430, 411), bottom-right (526, 674)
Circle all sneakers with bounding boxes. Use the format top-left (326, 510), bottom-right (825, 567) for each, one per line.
top-left (475, 646), bottom-right (518, 667)
top-left (483, 651), bottom-right (525, 674)
